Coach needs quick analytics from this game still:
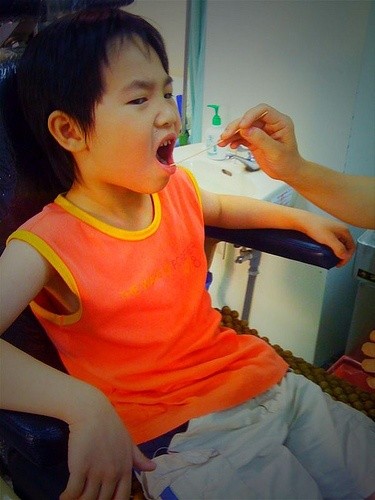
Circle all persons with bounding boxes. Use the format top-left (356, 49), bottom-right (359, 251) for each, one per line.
top-left (216, 102), bottom-right (374, 230)
top-left (0, 6), bottom-right (375, 500)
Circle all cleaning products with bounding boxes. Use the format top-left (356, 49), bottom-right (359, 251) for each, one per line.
top-left (204, 104), bottom-right (229, 161)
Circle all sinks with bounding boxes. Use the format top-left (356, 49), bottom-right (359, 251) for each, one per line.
top-left (179, 140), bottom-right (295, 207)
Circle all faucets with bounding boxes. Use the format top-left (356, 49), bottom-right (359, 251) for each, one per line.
top-left (226, 144), bottom-right (260, 172)
